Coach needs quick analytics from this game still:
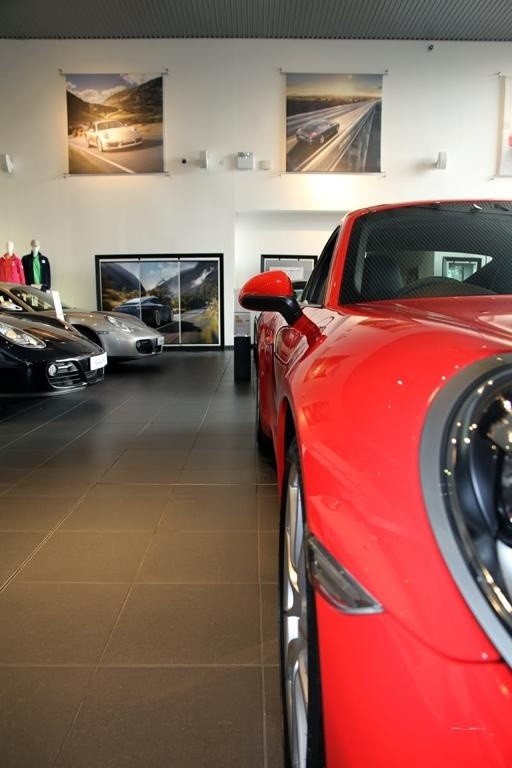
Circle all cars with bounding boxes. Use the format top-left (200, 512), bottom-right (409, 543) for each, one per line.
top-left (0, 309), bottom-right (110, 399)
top-left (295, 118), bottom-right (340, 144)
top-left (112, 293), bottom-right (175, 327)
top-left (0, 278), bottom-right (166, 363)
top-left (83, 118), bottom-right (144, 154)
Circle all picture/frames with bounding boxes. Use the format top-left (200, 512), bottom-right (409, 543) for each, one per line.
top-left (442, 255), bottom-right (483, 287)
top-left (259, 251), bottom-right (320, 300)
top-left (93, 251), bottom-right (226, 351)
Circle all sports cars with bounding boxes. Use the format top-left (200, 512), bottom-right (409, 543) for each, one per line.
top-left (233, 196), bottom-right (512, 768)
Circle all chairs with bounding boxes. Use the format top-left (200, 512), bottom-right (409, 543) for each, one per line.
top-left (355, 256), bottom-right (410, 302)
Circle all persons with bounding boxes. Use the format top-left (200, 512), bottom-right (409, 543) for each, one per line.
top-left (21, 240), bottom-right (51, 290)
top-left (0, 239), bottom-right (26, 285)
